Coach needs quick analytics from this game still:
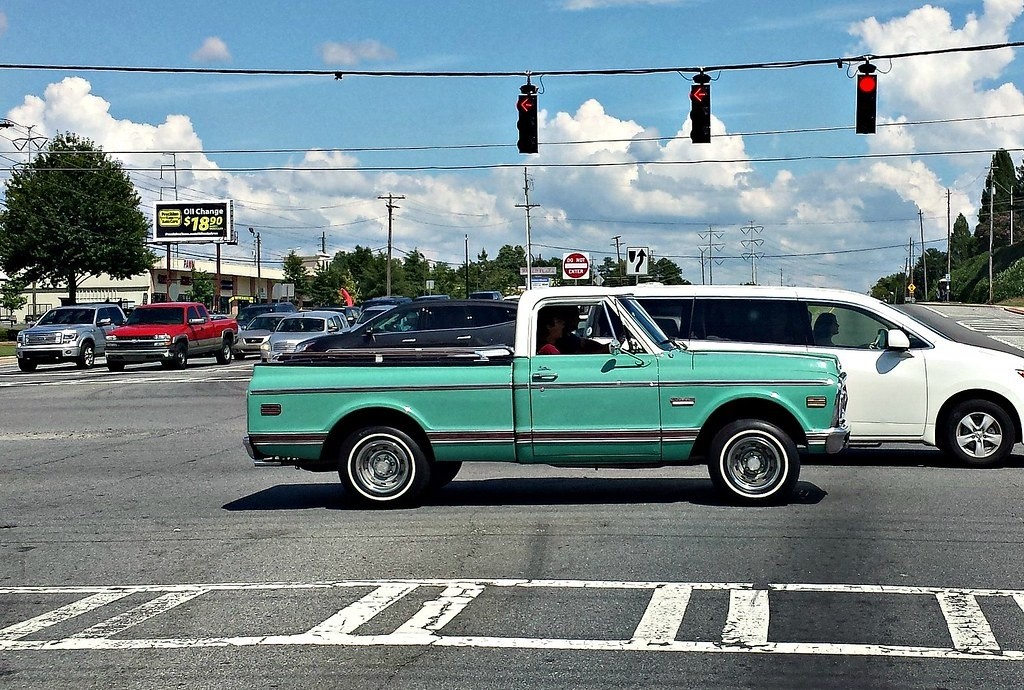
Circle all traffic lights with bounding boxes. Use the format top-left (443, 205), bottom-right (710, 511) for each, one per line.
top-left (516, 95), bottom-right (538, 153)
top-left (856, 74), bottom-right (876, 132)
top-left (689, 84), bottom-right (711, 144)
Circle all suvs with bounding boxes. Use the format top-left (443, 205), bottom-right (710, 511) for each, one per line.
top-left (16, 304), bottom-right (128, 371)
top-left (235, 292), bottom-right (521, 362)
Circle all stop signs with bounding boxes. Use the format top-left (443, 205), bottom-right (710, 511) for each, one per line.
top-left (562, 252), bottom-right (589, 280)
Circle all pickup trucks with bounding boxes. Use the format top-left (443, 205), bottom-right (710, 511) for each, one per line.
top-left (244, 287), bottom-right (850, 506)
top-left (105, 302), bottom-right (239, 370)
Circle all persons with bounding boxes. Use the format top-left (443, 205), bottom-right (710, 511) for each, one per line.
top-left (813, 313), bottom-right (840, 346)
top-left (536, 306), bottom-right (610, 354)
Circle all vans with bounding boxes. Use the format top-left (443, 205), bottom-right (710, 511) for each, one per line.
top-left (585, 286), bottom-right (1024, 466)
